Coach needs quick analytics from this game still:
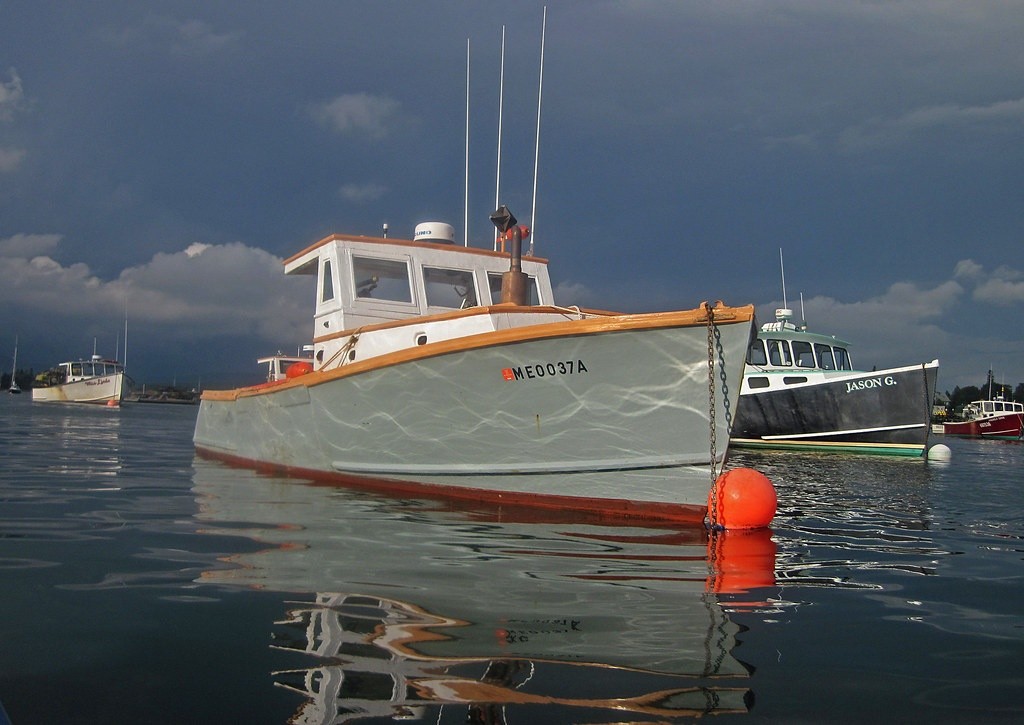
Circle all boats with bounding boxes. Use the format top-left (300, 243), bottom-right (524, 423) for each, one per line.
top-left (32, 354), bottom-right (133, 405)
top-left (728, 247), bottom-right (939, 457)
top-left (9, 385), bottom-right (22, 394)
top-left (943, 363), bottom-right (1024, 440)
top-left (192, 6), bottom-right (755, 526)
